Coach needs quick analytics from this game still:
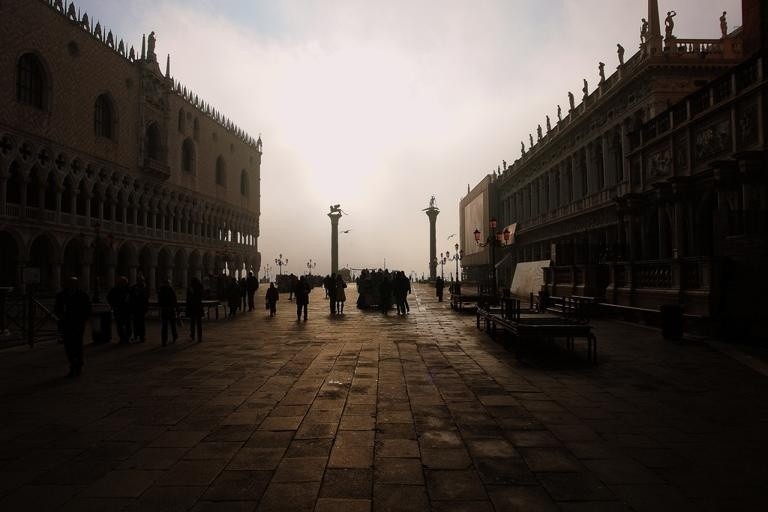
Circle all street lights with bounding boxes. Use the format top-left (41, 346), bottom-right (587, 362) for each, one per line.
top-left (306, 258), bottom-right (317, 275)
top-left (473, 218), bottom-right (511, 306)
top-left (263, 263), bottom-right (272, 277)
top-left (446, 243), bottom-right (464, 295)
top-left (275, 253), bottom-right (289, 275)
top-left (437, 253), bottom-right (446, 279)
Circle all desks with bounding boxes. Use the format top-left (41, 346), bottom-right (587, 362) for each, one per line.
top-left (450, 282), bottom-right (597, 368)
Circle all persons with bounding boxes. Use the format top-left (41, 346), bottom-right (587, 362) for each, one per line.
top-left (105, 276), bottom-right (133, 347)
top-left (486, 17), bottom-right (649, 181)
top-left (720, 10), bottom-right (729, 38)
top-left (319, 274), bottom-right (330, 299)
top-left (324, 272), bottom-right (337, 314)
top-left (126, 273), bottom-right (150, 344)
top-left (51, 275), bottom-right (91, 387)
top-left (286, 273), bottom-right (296, 300)
top-left (200, 269), bottom-right (259, 319)
top-left (355, 267), bottom-right (411, 316)
top-left (146, 30), bottom-right (157, 59)
top-left (434, 275), bottom-right (444, 302)
top-left (664, 10), bottom-right (677, 35)
top-left (185, 280), bottom-right (207, 343)
top-left (293, 279), bottom-right (310, 320)
top-left (334, 273), bottom-right (347, 316)
top-left (265, 282), bottom-right (279, 318)
top-left (156, 279), bottom-right (178, 347)
